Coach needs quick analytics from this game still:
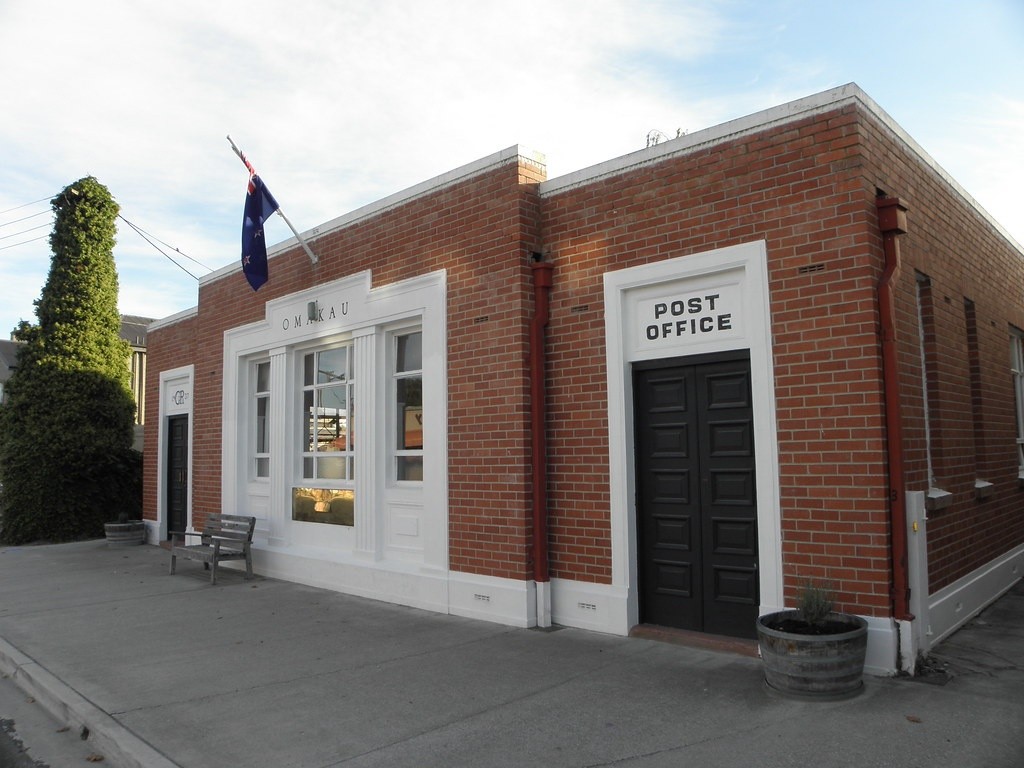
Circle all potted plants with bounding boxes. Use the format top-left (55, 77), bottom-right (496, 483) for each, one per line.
top-left (755, 570), bottom-right (868, 702)
top-left (103, 511), bottom-right (145, 545)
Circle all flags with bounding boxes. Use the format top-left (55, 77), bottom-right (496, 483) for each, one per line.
top-left (238, 151), bottom-right (280, 293)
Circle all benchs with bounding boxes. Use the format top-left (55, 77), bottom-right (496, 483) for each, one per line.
top-left (168, 513), bottom-right (255, 585)
